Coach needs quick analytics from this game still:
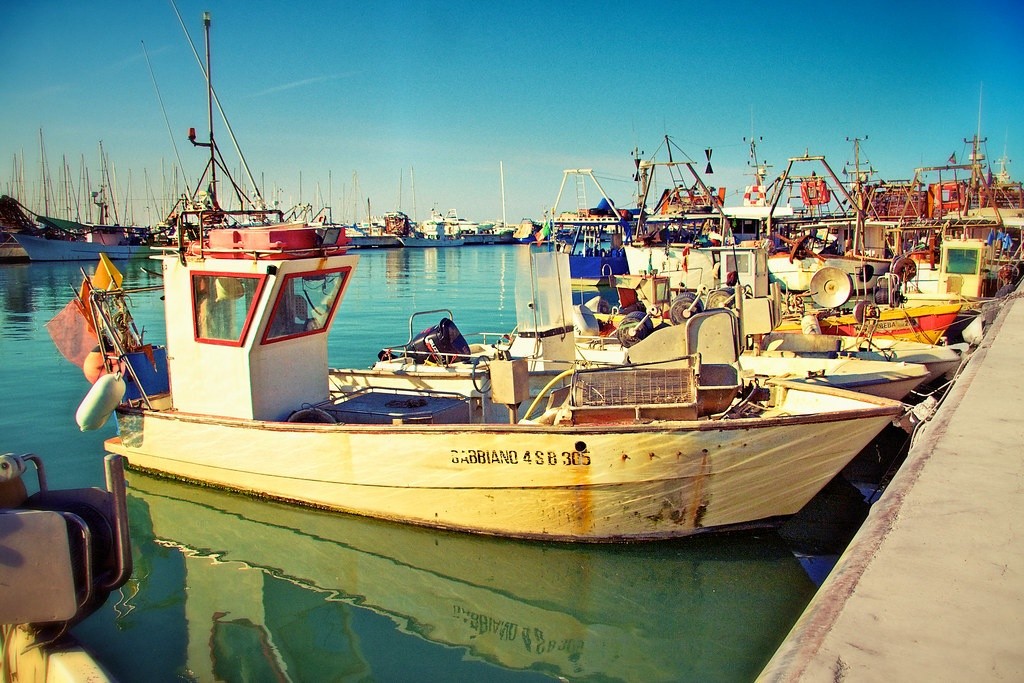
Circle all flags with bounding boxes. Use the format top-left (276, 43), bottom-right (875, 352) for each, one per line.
top-left (948, 152), bottom-right (956, 165)
top-left (534, 224), bottom-right (550, 247)
top-left (988, 230), bottom-right (1013, 251)
top-left (47, 253), bottom-right (124, 366)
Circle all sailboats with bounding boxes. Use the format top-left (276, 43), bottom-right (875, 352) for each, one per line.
top-left (0, 91), bottom-right (1023, 402)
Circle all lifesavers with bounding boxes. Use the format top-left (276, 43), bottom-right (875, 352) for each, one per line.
top-left (889, 255), bottom-right (918, 283)
top-left (742, 185), bottom-right (766, 207)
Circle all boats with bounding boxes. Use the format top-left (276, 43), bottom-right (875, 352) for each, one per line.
top-left (73, 11), bottom-right (907, 549)
top-left (0, 451), bottom-right (136, 683)
top-left (119, 464), bottom-right (820, 682)
